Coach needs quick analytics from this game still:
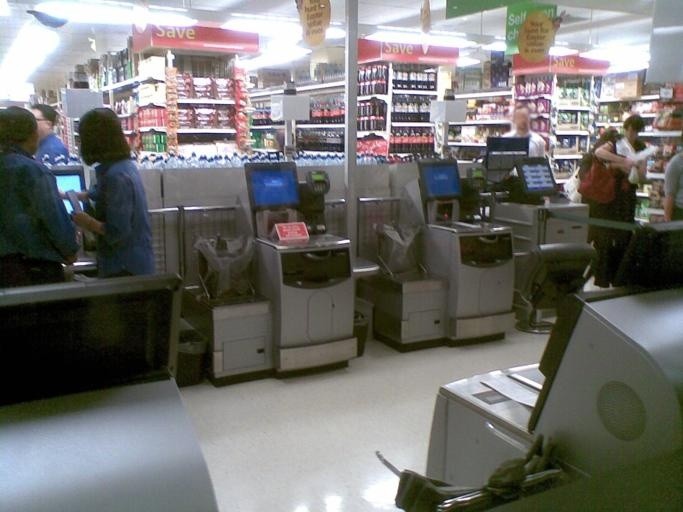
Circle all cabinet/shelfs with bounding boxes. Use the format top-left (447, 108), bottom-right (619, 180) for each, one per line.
top-left (357, 269), bottom-right (451, 352)
top-left (186, 287), bottom-right (274, 390)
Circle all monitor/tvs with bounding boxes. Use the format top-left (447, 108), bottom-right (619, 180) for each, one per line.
top-left (515, 157), bottom-right (557, 195)
top-left (487, 137), bottom-right (529, 154)
top-left (50, 165), bottom-right (85, 213)
top-left (243, 161), bottom-right (303, 209)
top-left (612, 217), bottom-right (683, 292)
top-left (418, 159), bottom-right (464, 200)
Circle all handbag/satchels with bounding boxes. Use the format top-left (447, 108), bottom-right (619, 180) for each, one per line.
top-left (577, 162), bottom-right (615, 203)
top-left (562, 166), bottom-right (581, 203)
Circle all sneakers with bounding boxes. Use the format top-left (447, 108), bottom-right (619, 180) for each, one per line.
top-left (593, 279), bottom-right (620, 288)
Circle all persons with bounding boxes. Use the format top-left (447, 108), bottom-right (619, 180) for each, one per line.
top-left (0, 107), bottom-right (77, 283)
top-left (29, 105), bottom-right (67, 164)
top-left (56, 107), bottom-right (152, 276)
top-left (499, 104), bottom-right (682, 286)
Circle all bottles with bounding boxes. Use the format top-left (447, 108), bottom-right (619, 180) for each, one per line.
top-left (357, 62), bottom-right (388, 131)
top-left (43, 151), bottom-right (439, 169)
top-left (251, 101), bottom-right (284, 125)
top-left (390, 66), bottom-right (435, 154)
top-left (296, 100), bottom-right (344, 152)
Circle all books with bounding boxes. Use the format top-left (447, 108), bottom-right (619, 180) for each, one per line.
top-left (65, 189), bottom-right (94, 243)
